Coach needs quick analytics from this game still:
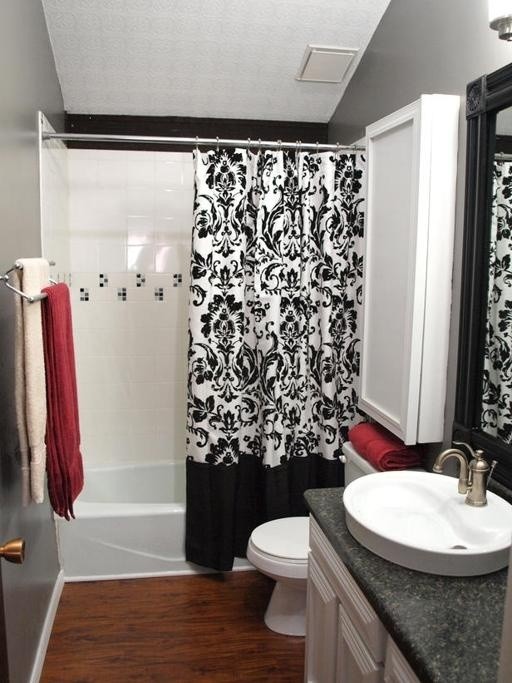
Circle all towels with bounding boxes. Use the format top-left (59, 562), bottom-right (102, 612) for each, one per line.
top-left (303, 519), bottom-right (418, 683)
top-left (17, 258), bottom-right (84, 523)
top-left (350, 424), bottom-right (416, 471)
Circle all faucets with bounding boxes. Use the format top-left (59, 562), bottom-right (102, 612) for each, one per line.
top-left (432, 440), bottom-right (491, 508)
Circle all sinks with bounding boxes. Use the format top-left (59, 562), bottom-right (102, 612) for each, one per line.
top-left (342, 470), bottom-right (512, 576)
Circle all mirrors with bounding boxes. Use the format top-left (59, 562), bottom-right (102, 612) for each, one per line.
top-left (451, 63), bottom-right (512, 501)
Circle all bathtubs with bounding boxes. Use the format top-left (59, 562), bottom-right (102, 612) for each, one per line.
top-left (53, 459), bottom-right (257, 583)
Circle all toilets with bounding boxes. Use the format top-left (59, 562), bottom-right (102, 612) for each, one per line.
top-left (245, 440), bottom-right (427, 638)
